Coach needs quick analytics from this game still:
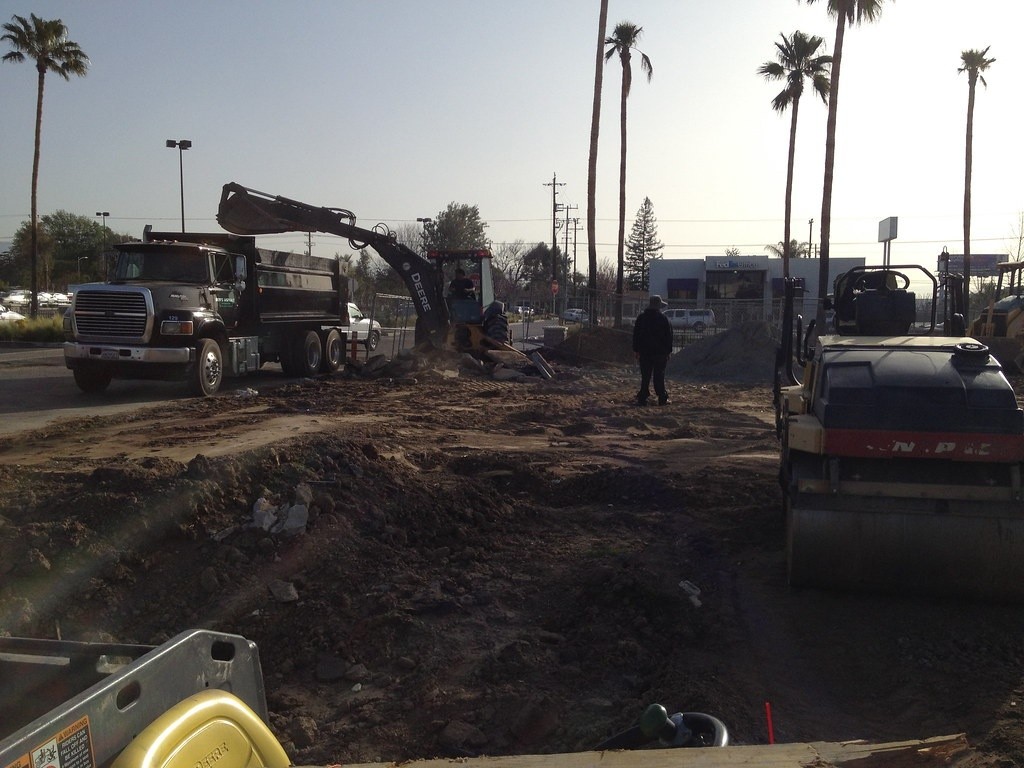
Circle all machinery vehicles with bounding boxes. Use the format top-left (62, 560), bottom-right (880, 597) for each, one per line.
top-left (215, 181), bottom-right (510, 348)
top-left (771, 265), bottom-right (1024, 599)
top-left (965, 261), bottom-right (1024, 372)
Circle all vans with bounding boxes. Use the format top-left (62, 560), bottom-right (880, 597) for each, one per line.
top-left (661, 309), bottom-right (717, 333)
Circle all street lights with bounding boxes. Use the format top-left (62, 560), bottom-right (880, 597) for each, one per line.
top-left (96, 212), bottom-right (109, 282)
top-left (78, 256), bottom-right (88, 284)
top-left (417, 217), bottom-right (431, 258)
top-left (166, 139), bottom-right (192, 233)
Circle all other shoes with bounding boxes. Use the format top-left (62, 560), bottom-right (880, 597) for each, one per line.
top-left (636, 394), bottom-right (649, 405)
top-left (658, 398), bottom-right (672, 406)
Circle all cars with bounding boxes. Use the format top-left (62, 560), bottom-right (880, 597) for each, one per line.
top-left (563, 308), bottom-right (589, 323)
top-left (0, 289), bottom-right (72, 327)
top-left (514, 306), bottom-right (535, 316)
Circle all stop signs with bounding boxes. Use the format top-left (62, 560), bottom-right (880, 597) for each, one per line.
top-left (552, 280), bottom-right (559, 292)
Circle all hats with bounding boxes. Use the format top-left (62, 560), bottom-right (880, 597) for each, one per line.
top-left (646, 295), bottom-right (668, 310)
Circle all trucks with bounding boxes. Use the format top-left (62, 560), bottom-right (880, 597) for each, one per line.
top-left (62, 225), bottom-right (349, 396)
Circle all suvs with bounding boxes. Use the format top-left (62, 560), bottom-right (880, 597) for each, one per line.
top-left (321, 302), bottom-right (382, 351)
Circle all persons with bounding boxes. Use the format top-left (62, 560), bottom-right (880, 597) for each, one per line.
top-left (634, 296), bottom-right (674, 406)
top-left (450, 269), bottom-right (476, 299)
